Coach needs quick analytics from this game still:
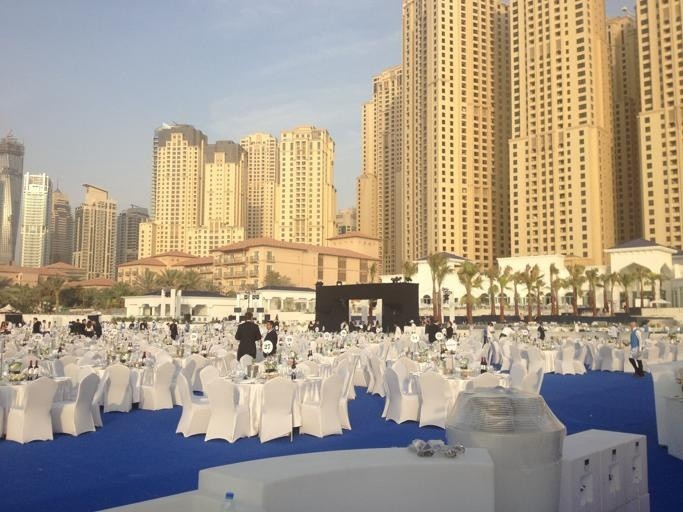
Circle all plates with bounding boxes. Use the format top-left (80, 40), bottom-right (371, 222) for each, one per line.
top-left (228, 373), bottom-right (323, 383)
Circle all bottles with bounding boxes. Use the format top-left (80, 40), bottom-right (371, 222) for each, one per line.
top-left (315, 338), bottom-right (361, 356)
top-left (196, 336), bottom-right (201, 345)
top-left (27, 360), bottom-right (34, 381)
top-left (290, 358), bottom-right (296, 379)
top-left (368, 333), bottom-right (392, 342)
top-left (180, 331), bottom-right (183, 337)
top-left (520, 334), bottom-right (553, 348)
top-left (21, 337), bottom-right (26, 347)
top-left (594, 335), bottom-right (597, 340)
top-left (437, 347), bottom-right (487, 379)
top-left (175, 344), bottom-right (207, 358)
top-left (219, 490), bottom-right (238, 511)
top-left (206, 330), bottom-right (224, 344)
top-left (180, 338), bottom-right (184, 347)
top-left (140, 351), bottom-right (147, 366)
top-left (34, 359), bottom-right (39, 380)
top-left (120, 334), bottom-right (132, 353)
top-left (308, 349), bottom-right (313, 361)
top-left (53, 334), bottom-right (81, 360)
top-left (145, 327), bottom-right (167, 343)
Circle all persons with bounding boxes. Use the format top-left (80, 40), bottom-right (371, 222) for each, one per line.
top-left (275, 321), bottom-right (287, 334)
top-left (234, 310), bottom-right (262, 361)
top-left (611, 321), bottom-right (620, 340)
top-left (263, 320), bottom-right (278, 359)
top-left (537, 319), bottom-right (548, 340)
top-left (68, 318), bottom-right (102, 339)
top-left (422, 317), bottom-right (458, 343)
top-left (629, 318), bottom-right (646, 377)
top-left (120, 319), bottom-right (157, 331)
top-left (484, 322), bottom-right (531, 344)
top-left (170, 319), bottom-right (190, 340)
top-left (28, 317), bottom-right (57, 335)
top-left (213, 320), bottom-right (224, 332)
top-left (337, 318), bottom-right (417, 342)
top-left (1, 319), bottom-right (25, 335)
top-left (308, 320), bottom-right (325, 334)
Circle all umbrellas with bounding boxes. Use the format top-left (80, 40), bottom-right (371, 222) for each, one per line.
top-left (0, 304), bottom-right (22, 315)
